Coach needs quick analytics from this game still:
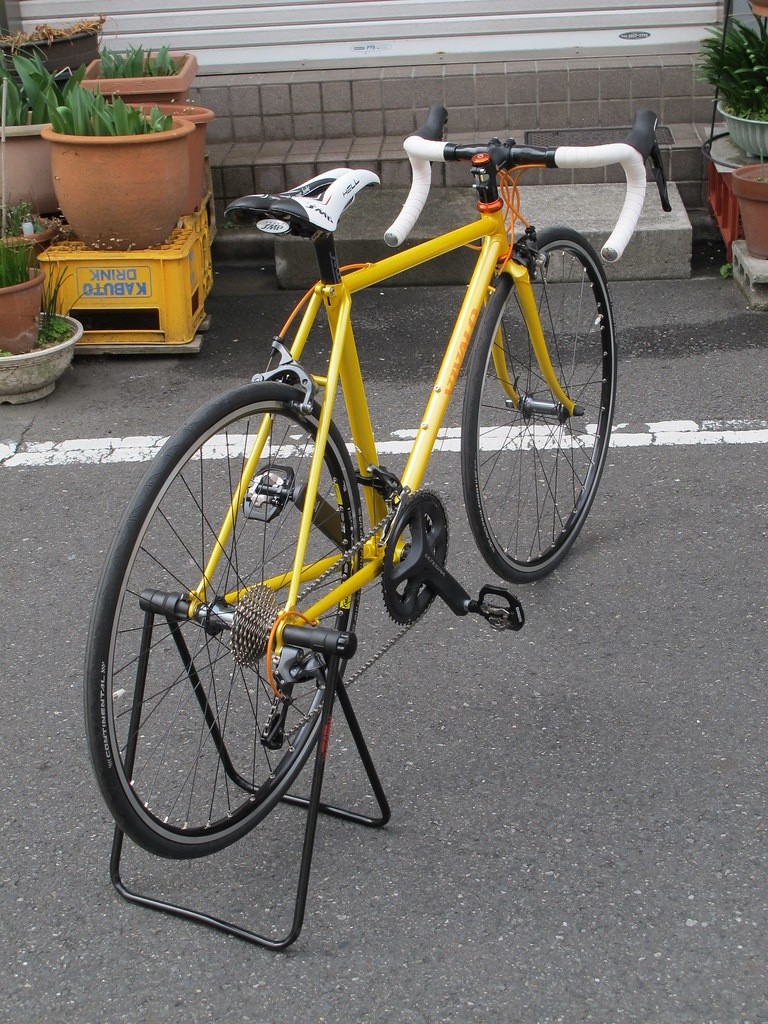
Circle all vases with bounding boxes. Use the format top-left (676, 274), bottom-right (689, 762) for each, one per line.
top-left (732, 163), bottom-right (768, 260)
top-left (0, 25), bottom-right (99, 111)
top-left (126, 104), bottom-right (215, 215)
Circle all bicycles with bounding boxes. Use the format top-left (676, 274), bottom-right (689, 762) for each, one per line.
top-left (80, 104), bottom-right (671, 953)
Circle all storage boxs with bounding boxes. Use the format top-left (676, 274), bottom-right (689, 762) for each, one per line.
top-left (36, 228), bottom-right (205, 345)
top-left (178, 206), bottom-right (214, 300)
top-left (707, 160), bottom-right (744, 262)
top-left (203, 155), bottom-right (216, 245)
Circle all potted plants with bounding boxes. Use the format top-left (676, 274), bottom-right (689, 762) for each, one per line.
top-left (690, 1), bottom-right (768, 158)
top-left (0, 198), bottom-right (58, 269)
top-left (80, 55), bottom-right (199, 104)
top-left (0, 244), bottom-right (106, 404)
top-left (0, 49), bottom-right (86, 215)
top-left (40, 81), bottom-right (197, 250)
top-left (0, 232), bottom-right (46, 356)
